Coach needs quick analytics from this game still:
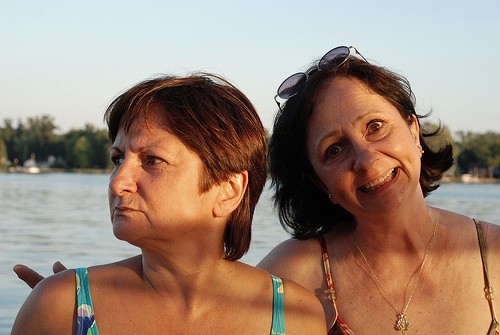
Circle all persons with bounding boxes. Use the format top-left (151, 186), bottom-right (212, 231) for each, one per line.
top-left (13, 43), bottom-right (500, 335)
top-left (10, 70), bottom-right (328, 335)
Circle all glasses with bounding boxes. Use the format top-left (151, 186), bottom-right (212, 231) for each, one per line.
top-left (274, 46), bottom-right (368, 115)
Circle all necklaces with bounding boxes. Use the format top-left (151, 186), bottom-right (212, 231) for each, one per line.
top-left (351, 218), bottom-right (437, 334)
